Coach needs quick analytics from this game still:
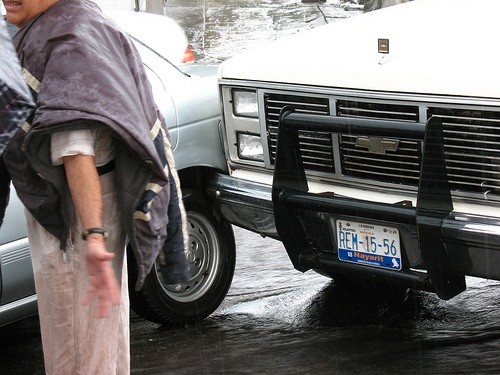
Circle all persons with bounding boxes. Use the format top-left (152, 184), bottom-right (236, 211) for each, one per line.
top-left (0, 0), bottom-right (187, 375)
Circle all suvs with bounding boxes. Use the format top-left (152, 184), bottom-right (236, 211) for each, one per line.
top-left (208, 1), bottom-right (500, 300)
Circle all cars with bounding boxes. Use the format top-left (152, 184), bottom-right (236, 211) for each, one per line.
top-left (0, 11), bottom-right (236, 335)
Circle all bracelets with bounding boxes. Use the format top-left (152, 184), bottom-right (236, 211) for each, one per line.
top-left (82, 227), bottom-right (106, 240)
